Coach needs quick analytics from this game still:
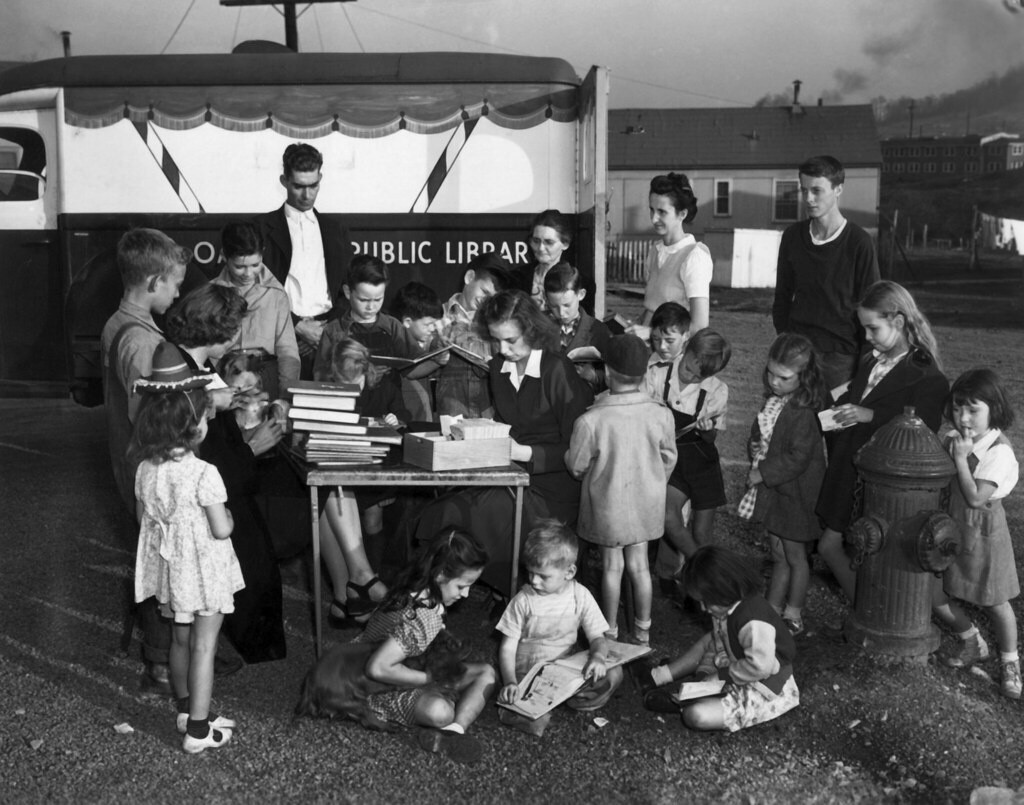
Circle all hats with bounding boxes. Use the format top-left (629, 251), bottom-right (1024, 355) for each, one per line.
top-left (132, 340), bottom-right (213, 394)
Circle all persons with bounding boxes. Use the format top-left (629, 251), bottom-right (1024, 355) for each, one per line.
top-left (738, 333), bottom-right (830, 638)
top-left (628, 543), bottom-right (800, 731)
top-left (932, 368), bottom-right (1024, 698)
top-left (260, 143), bottom-right (354, 381)
top-left (493, 518), bottom-right (623, 738)
top-left (127, 342), bottom-right (238, 751)
top-left (773, 156), bottom-right (881, 424)
top-left (101, 220), bottom-right (389, 696)
top-left (817, 280), bottom-right (949, 601)
top-left (350, 526), bottom-right (500, 764)
top-left (623, 172), bottom-right (713, 339)
top-left (315, 210), bottom-right (732, 652)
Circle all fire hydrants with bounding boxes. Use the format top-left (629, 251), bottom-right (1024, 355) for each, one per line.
top-left (838, 404), bottom-right (970, 668)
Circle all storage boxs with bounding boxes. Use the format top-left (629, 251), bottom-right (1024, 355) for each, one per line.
top-left (403, 432), bottom-right (511, 471)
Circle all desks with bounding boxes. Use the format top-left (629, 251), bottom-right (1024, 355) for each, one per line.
top-left (305, 454), bottom-right (530, 663)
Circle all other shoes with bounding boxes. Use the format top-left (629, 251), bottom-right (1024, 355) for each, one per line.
top-left (680, 600), bottom-right (703, 623)
top-left (629, 661), bottom-right (657, 694)
top-left (659, 577), bottom-right (680, 597)
top-left (644, 688), bottom-right (681, 714)
top-left (823, 622), bottom-right (843, 637)
top-left (182, 722), bottom-right (232, 753)
top-left (782, 617), bottom-right (804, 635)
top-left (625, 633), bottom-right (650, 648)
top-left (419, 728), bottom-right (482, 763)
top-left (150, 664), bottom-right (171, 683)
top-left (176, 713), bottom-right (237, 734)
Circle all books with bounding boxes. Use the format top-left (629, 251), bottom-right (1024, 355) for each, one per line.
top-left (288, 380), bottom-right (402, 471)
top-left (495, 639), bottom-right (654, 719)
top-left (566, 346), bottom-right (604, 363)
top-left (603, 313), bottom-right (651, 348)
top-left (370, 345), bottom-right (456, 370)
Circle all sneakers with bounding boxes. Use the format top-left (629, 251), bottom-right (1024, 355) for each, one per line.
top-left (999, 661), bottom-right (1023, 700)
top-left (948, 633), bottom-right (990, 668)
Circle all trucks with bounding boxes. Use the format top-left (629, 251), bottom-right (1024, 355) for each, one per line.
top-left (0, 40), bottom-right (612, 409)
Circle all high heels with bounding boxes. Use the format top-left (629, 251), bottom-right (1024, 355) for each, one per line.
top-left (346, 577), bottom-right (390, 615)
top-left (330, 599), bottom-right (370, 629)
